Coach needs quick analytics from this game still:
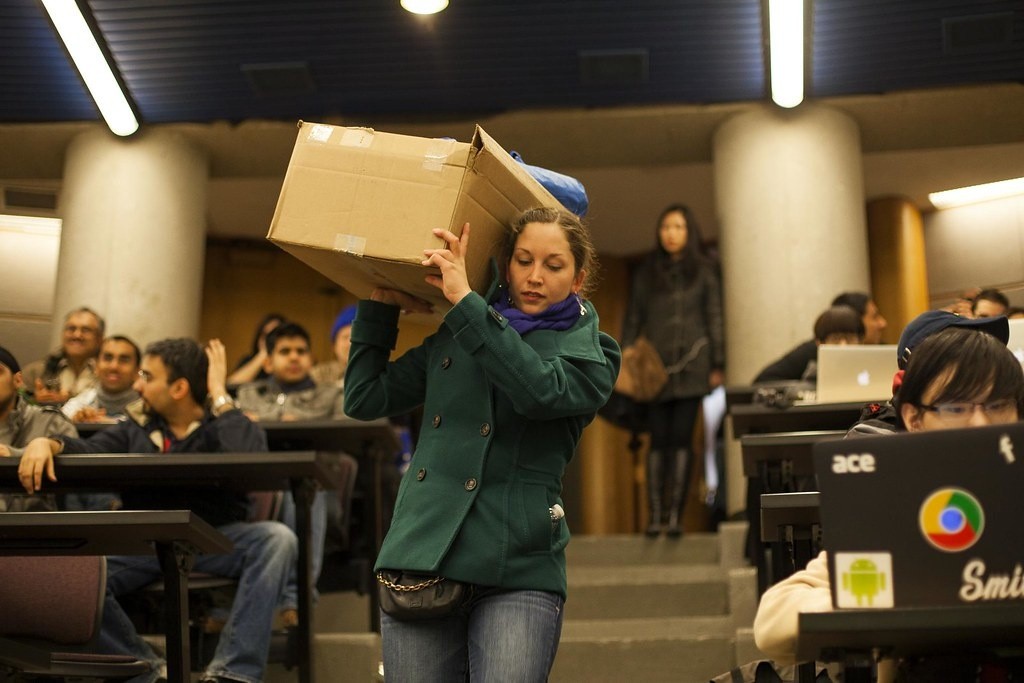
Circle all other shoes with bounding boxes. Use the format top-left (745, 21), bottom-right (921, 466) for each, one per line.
top-left (202, 615), bottom-right (224, 634)
top-left (280, 608), bottom-right (299, 629)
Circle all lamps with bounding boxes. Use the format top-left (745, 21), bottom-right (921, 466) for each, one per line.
top-left (42, 0), bottom-right (139, 136)
top-left (770, 0), bottom-right (804, 108)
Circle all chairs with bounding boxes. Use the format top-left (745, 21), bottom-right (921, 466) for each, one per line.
top-left (141, 489), bottom-right (285, 672)
top-left (0, 553), bottom-right (149, 683)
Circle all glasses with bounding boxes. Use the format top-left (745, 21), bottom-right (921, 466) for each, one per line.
top-left (918, 401), bottom-right (1013, 427)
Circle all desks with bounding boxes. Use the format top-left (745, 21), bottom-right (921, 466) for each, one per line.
top-left (725, 383), bottom-right (1024, 683)
top-left (0, 420), bottom-right (403, 683)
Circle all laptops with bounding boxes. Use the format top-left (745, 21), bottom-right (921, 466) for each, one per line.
top-left (812, 420), bottom-right (1024, 612)
top-left (794, 343), bottom-right (901, 407)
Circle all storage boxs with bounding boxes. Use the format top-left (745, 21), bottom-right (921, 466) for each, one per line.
top-left (268, 119), bottom-right (578, 315)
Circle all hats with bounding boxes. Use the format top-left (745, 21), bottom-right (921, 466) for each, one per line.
top-left (331, 307), bottom-right (356, 344)
top-left (898, 311), bottom-right (1009, 370)
top-left (0, 348), bottom-right (20, 374)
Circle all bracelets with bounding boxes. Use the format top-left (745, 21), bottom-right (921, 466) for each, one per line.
top-left (211, 395), bottom-right (234, 413)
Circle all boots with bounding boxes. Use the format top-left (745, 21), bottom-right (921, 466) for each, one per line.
top-left (664, 451), bottom-right (694, 535)
top-left (644, 452), bottom-right (664, 536)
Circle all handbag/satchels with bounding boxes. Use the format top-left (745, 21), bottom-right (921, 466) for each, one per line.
top-left (376, 568), bottom-right (468, 622)
top-left (700, 385), bottom-right (726, 506)
top-left (614, 337), bottom-right (669, 401)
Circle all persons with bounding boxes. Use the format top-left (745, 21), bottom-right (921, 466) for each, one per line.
top-left (621, 205), bottom-right (728, 540)
top-left (342, 206), bottom-right (623, 683)
top-left (752, 285), bottom-right (1024, 683)
top-left (0, 304), bottom-right (412, 683)
